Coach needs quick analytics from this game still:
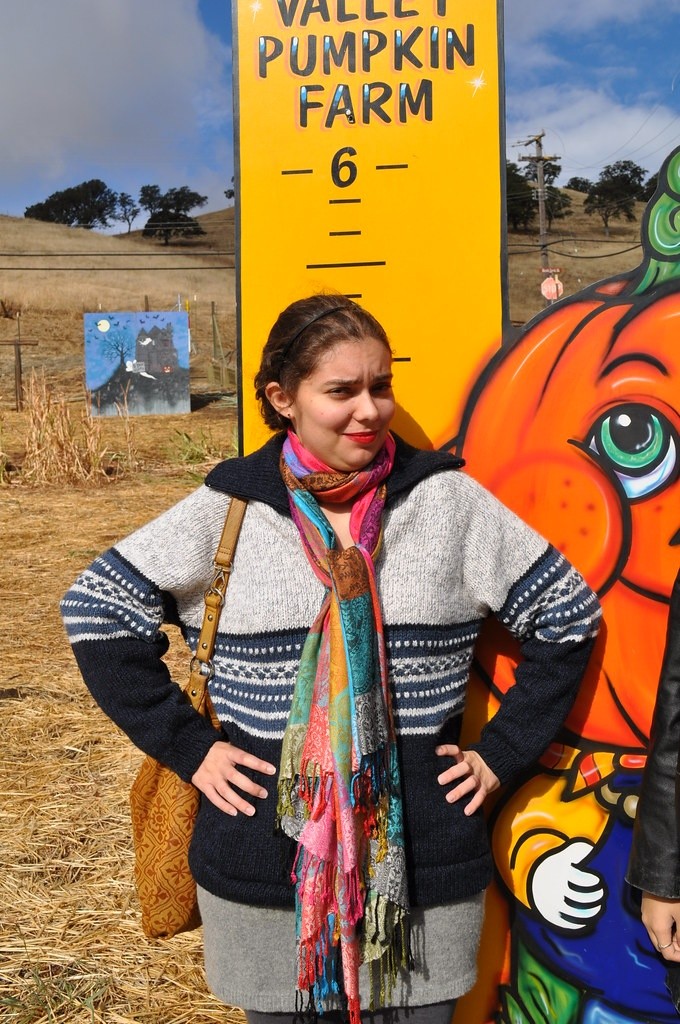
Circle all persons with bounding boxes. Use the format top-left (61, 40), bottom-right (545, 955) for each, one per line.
top-left (624, 567), bottom-right (680, 1014)
top-left (54, 291), bottom-right (604, 1024)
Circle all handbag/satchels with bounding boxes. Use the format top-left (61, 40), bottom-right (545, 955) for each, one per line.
top-left (129, 678), bottom-right (221, 943)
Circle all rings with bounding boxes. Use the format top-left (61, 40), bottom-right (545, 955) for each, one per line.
top-left (658, 941), bottom-right (672, 949)
